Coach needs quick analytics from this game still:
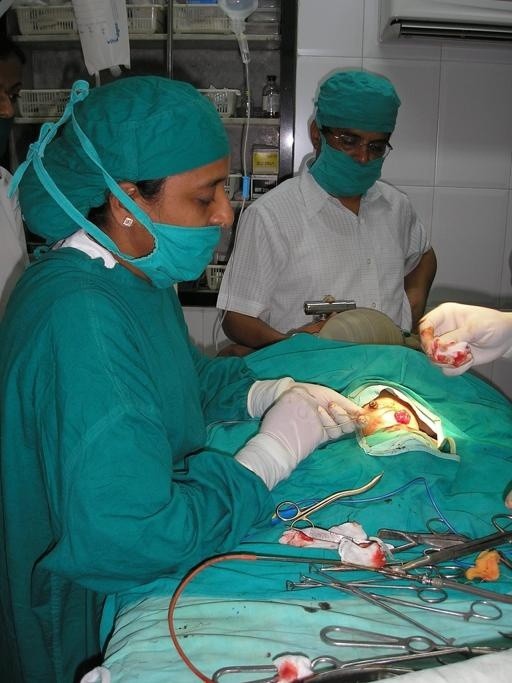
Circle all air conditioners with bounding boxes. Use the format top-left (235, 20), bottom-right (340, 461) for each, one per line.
top-left (378, 0), bottom-right (512, 46)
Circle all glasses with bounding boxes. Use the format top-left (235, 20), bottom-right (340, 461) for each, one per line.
top-left (325, 127), bottom-right (393, 157)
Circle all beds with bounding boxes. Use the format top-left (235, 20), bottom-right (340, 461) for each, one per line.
top-left (91, 335), bottom-right (512, 683)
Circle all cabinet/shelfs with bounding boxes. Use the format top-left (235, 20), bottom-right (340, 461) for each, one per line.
top-left (180, 307), bottom-right (236, 357)
top-left (9, 32), bottom-right (282, 212)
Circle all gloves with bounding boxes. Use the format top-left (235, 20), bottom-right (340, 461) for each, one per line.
top-left (417, 300), bottom-right (512, 378)
top-left (233, 388), bottom-right (325, 490)
top-left (248, 379), bottom-right (364, 440)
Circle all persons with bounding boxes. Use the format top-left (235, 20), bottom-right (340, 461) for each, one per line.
top-left (0, 75), bottom-right (369, 683)
top-left (0, 39), bottom-right (30, 308)
top-left (419, 301), bottom-right (512, 511)
top-left (216, 69), bottom-right (437, 351)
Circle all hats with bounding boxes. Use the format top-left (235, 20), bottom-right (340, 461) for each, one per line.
top-left (314, 71), bottom-right (397, 135)
top-left (20, 76), bottom-right (230, 238)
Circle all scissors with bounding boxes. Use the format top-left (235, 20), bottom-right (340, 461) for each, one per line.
top-left (287, 515), bottom-right (512, 622)
top-left (276, 474), bottom-right (382, 529)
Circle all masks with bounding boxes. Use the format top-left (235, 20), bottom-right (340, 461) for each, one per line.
top-left (131, 221), bottom-right (220, 288)
top-left (309, 147), bottom-right (382, 196)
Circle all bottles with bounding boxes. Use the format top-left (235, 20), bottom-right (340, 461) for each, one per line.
top-left (260, 75), bottom-right (281, 118)
top-left (214, 255), bottom-right (227, 280)
